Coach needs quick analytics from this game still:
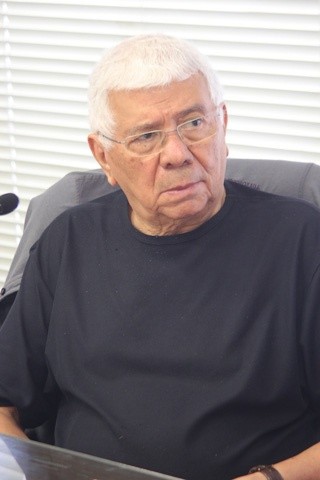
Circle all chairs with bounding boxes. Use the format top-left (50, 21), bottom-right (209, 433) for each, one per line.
top-left (0, 158), bottom-right (320, 326)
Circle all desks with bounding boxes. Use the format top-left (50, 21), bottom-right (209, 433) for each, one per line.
top-left (0, 433), bottom-right (185, 480)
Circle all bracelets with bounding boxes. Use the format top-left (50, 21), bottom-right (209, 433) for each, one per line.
top-left (248, 465), bottom-right (285, 480)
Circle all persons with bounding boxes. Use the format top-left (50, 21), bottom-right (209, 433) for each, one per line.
top-left (1, 33), bottom-right (319, 480)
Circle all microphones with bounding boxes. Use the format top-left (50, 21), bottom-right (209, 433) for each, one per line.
top-left (0, 193), bottom-right (19, 216)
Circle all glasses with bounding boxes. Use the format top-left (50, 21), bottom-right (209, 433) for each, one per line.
top-left (96, 106), bottom-right (222, 158)
